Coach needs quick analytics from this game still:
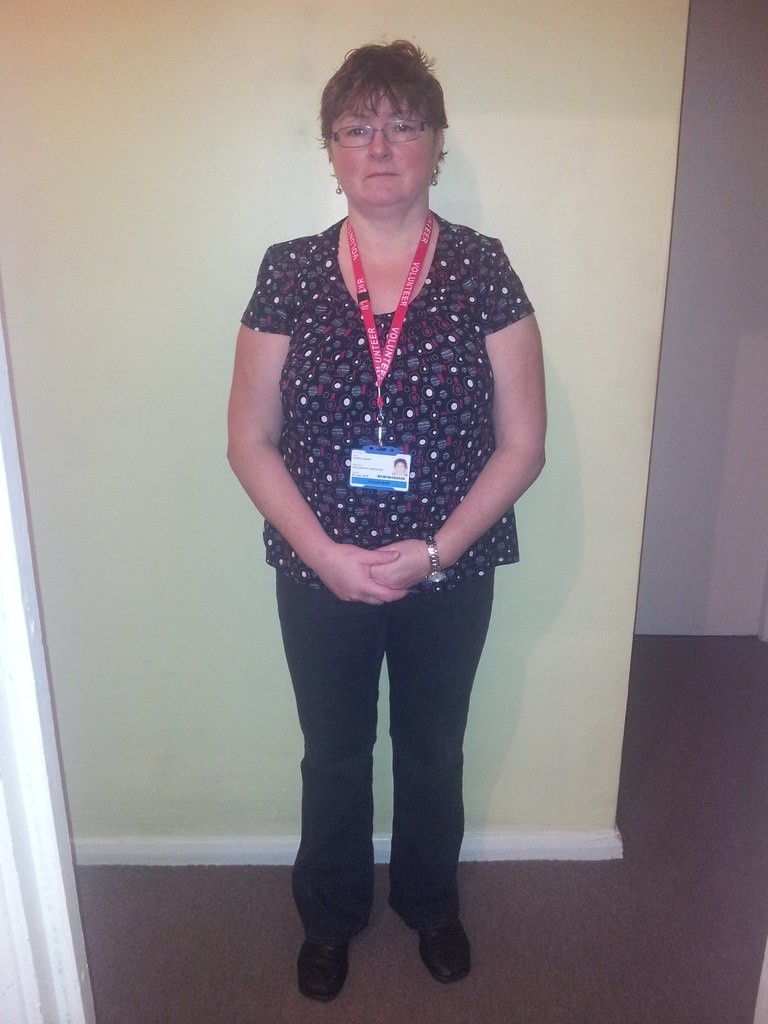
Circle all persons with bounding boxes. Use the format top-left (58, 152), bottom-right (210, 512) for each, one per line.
top-left (215, 37), bottom-right (551, 1003)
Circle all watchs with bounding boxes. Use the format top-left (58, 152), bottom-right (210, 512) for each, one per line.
top-left (423, 535), bottom-right (449, 584)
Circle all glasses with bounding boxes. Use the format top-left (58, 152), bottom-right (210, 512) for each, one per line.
top-left (331, 119), bottom-right (427, 148)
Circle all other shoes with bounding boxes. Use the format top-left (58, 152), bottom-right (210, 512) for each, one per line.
top-left (297, 936), bottom-right (348, 1000)
top-left (419, 916), bottom-right (471, 983)
